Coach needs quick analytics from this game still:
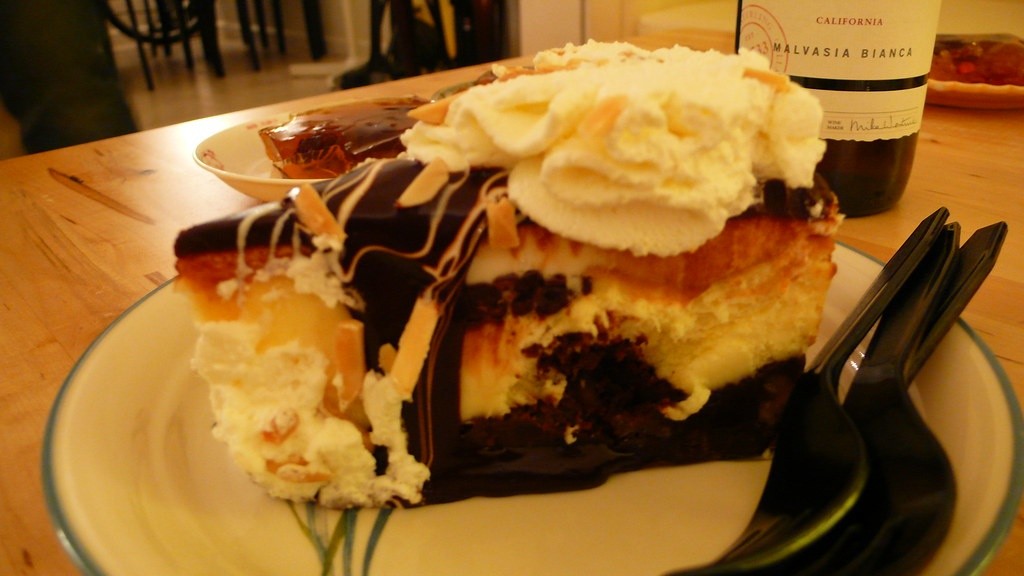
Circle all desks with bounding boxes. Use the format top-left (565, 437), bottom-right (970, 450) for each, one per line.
top-left (0, 51), bottom-right (1024, 576)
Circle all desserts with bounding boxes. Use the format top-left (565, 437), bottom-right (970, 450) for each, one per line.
top-left (173, 40), bottom-right (848, 507)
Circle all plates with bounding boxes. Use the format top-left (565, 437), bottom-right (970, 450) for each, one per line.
top-left (923, 71), bottom-right (1024, 110)
top-left (38, 239), bottom-right (1024, 576)
top-left (192, 95), bottom-right (423, 204)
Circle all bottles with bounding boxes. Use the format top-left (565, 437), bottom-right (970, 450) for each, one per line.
top-left (735, 0), bottom-right (942, 219)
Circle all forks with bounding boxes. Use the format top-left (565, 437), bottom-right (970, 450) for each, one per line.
top-left (665, 207), bottom-right (1008, 576)
